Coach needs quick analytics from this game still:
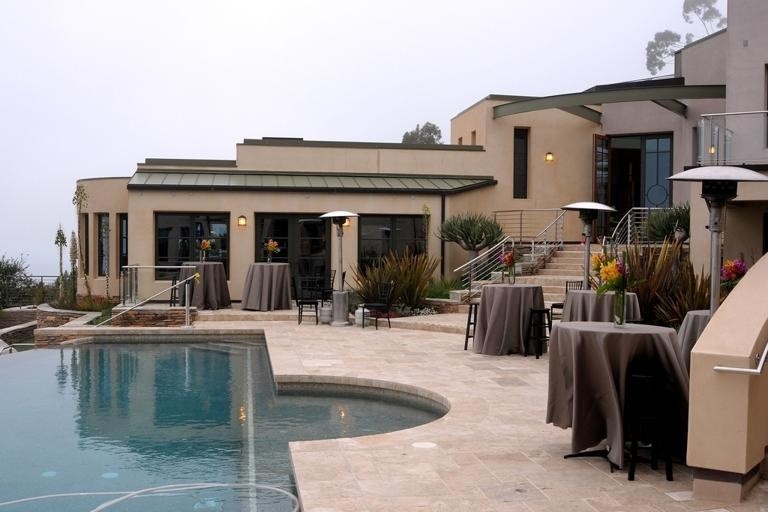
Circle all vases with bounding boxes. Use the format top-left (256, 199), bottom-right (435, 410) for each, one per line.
top-left (200, 250), bottom-right (205, 263)
top-left (266, 256), bottom-right (272, 264)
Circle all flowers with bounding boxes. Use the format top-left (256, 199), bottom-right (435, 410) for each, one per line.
top-left (590, 252), bottom-right (647, 324)
top-left (499, 252), bottom-right (515, 285)
top-left (199, 239), bottom-right (211, 251)
top-left (262, 239), bottom-right (281, 258)
top-left (718, 257), bottom-right (751, 295)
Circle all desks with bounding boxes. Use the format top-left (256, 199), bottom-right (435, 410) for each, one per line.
top-left (250, 262), bottom-right (289, 311)
top-left (181, 261), bottom-right (223, 310)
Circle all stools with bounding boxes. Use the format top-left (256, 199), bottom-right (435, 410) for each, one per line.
top-left (524, 308), bottom-right (552, 359)
top-left (626, 366), bottom-right (675, 480)
top-left (464, 297), bottom-right (480, 350)
top-left (170, 276), bottom-right (179, 306)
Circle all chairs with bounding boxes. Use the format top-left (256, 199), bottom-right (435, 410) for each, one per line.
top-left (292, 261), bottom-right (336, 325)
top-left (551, 280), bottom-right (583, 325)
top-left (362, 280), bottom-right (397, 331)
